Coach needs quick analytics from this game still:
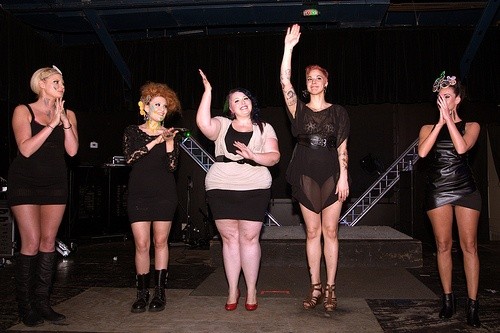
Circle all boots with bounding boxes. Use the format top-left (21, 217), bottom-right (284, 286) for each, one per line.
top-left (37, 249), bottom-right (66, 323)
top-left (131, 271), bottom-right (151, 312)
top-left (15, 251), bottom-right (45, 325)
top-left (466, 295), bottom-right (481, 329)
top-left (440, 290), bottom-right (458, 318)
top-left (149, 270), bottom-right (168, 312)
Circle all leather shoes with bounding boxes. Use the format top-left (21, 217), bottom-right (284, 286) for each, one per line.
top-left (244, 295), bottom-right (258, 311)
top-left (225, 289), bottom-right (240, 310)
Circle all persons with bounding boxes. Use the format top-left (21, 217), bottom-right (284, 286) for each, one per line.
top-left (121, 82), bottom-right (184, 314)
top-left (8, 65), bottom-right (80, 326)
top-left (196, 68), bottom-right (281, 311)
top-left (419, 70), bottom-right (482, 328)
top-left (279, 23), bottom-right (350, 312)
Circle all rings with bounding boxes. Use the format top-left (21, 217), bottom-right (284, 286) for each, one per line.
top-left (167, 135), bottom-right (171, 137)
top-left (439, 104), bottom-right (442, 108)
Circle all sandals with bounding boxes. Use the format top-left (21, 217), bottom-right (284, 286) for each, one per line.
top-left (324, 284), bottom-right (337, 312)
top-left (303, 282), bottom-right (325, 309)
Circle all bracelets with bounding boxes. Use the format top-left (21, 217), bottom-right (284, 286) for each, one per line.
top-left (64, 124), bottom-right (73, 130)
top-left (47, 123), bottom-right (55, 130)
